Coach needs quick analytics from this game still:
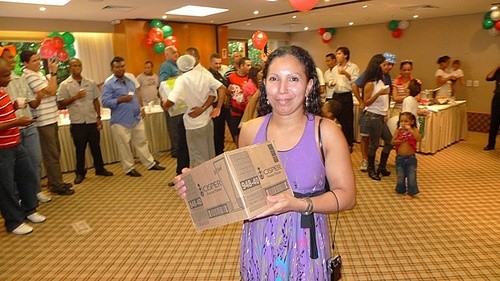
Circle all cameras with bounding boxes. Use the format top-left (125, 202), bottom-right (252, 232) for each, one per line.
top-left (327, 254), bottom-right (341, 281)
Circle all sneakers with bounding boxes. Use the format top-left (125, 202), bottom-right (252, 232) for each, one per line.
top-left (36, 192), bottom-right (52, 203)
top-left (359, 159), bottom-right (368, 171)
top-left (12, 223), bottom-right (33, 234)
top-left (26, 211), bottom-right (47, 223)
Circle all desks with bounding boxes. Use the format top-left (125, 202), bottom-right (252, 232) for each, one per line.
top-left (38, 105), bottom-right (176, 177)
top-left (353, 100), bottom-right (468, 154)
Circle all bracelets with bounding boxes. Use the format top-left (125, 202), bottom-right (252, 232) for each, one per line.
top-left (97, 116), bottom-right (101, 119)
top-left (51, 72), bottom-right (57, 76)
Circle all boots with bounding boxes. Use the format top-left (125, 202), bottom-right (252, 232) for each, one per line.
top-left (376, 152), bottom-right (391, 176)
top-left (368, 155), bottom-right (381, 180)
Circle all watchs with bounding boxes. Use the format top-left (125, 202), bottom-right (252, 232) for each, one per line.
top-left (301, 198), bottom-right (313, 216)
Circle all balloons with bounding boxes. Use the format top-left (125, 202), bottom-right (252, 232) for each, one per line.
top-left (144, 19), bottom-right (177, 53)
top-left (252, 31), bottom-right (268, 50)
top-left (289, 0), bottom-right (316, 11)
top-left (388, 20), bottom-right (409, 38)
top-left (482, 7), bottom-right (500, 36)
top-left (40, 32), bottom-right (76, 61)
top-left (319, 28), bottom-right (336, 44)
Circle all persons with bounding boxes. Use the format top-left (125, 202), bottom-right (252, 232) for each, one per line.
top-left (101, 57), bottom-right (166, 176)
top-left (0, 44), bottom-right (75, 206)
top-left (0, 58), bottom-right (47, 235)
top-left (351, 52), bottom-right (422, 199)
top-left (485, 67), bottom-right (500, 150)
top-left (316, 47), bottom-right (360, 154)
top-left (56, 59), bottom-right (114, 184)
top-left (157, 46), bottom-right (264, 186)
top-left (174, 46), bottom-right (356, 281)
top-left (435, 55), bottom-right (464, 99)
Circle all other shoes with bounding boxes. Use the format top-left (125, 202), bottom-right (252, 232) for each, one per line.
top-left (95, 170), bottom-right (113, 176)
top-left (172, 151), bottom-right (176, 158)
top-left (483, 145), bottom-right (495, 150)
top-left (57, 186), bottom-right (74, 195)
top-left (74, 175), bottom-right (85, 183)
top-left (149, 165), bottom-right (166, 170)
top-left (168, 181), bottom-right (176, 186)
top-left (126, 169), bottom-right (142, 177)
top-left (50, 183), bottom-right (73, 193)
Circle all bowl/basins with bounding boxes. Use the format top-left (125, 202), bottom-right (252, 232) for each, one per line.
top-left (390, 102), bottom-right (396, 108)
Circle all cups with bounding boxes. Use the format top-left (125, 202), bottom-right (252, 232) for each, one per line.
top-left (383, 85), bottom-right (390, 93)
top-left (17, 98), bottom-right (25, 108)
top-left (46, 74), bottom-right (50, 80)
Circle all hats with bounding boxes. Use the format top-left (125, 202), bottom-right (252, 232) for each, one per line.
top-left (0, 44), bottom-right (16, 58)
